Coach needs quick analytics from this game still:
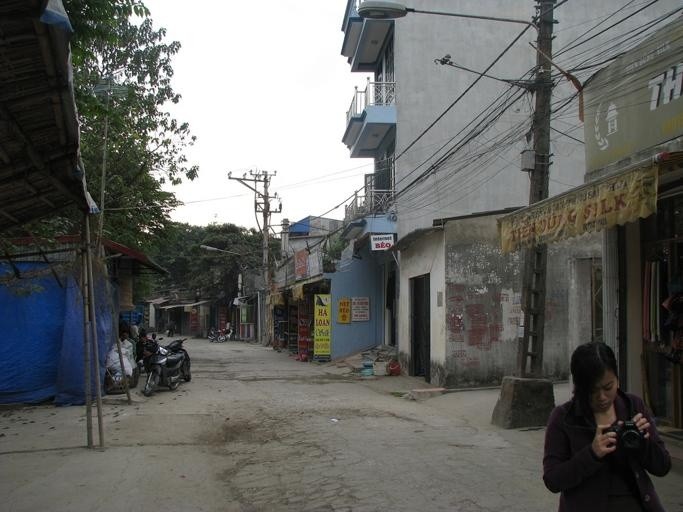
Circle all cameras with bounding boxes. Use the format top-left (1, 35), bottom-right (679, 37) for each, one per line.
top-left (603, 420), bottom-right (643, 455)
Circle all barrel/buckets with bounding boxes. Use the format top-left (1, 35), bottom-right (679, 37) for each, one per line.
top-left (372, 356), bottom-right (386, 376)
top-left (389, 359), bottom-right (401, 375)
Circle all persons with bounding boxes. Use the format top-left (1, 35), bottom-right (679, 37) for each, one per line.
top-left (538, 337), bottom-right (671, 511)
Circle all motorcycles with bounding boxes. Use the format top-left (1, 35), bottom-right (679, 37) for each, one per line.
top-left (208, 326), bottom-right (235, 343)
top-left (141, 338), bottom-right (192, 397)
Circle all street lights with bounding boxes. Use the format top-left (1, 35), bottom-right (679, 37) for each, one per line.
top-left (358, 0), bottom-right (554, 378)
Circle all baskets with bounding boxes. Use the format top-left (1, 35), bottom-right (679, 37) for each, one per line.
top-left (103, 374), bottom-right (130, 394)
